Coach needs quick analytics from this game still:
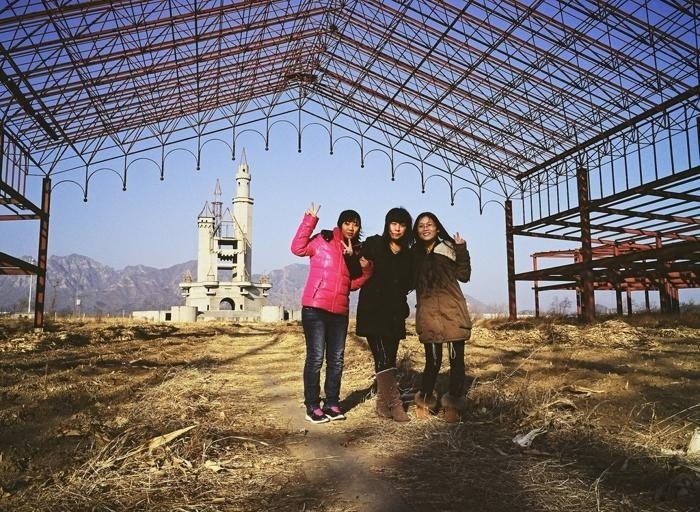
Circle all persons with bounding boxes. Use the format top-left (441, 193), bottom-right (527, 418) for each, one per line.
top-left (289, 201), bottom-right (376, 424)
top-left (410, 211), bottom-right (471, 422)
top-left (339, 207), bottom-right (418, 422)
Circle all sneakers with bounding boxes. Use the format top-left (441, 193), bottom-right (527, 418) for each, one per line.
top-left (322, 402), bottom-right (346, 420)
top-left (305, 408), bottom-right (330, 424)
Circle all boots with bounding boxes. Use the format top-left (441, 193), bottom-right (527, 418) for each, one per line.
top-left (414, 390), bottom-right (437, 421)
top-left (375, 367), bottom-right (410, 422)
top-left (375, 385), bottom-right (392, 418)
top-left (440, 392), bottom-right (467, 422)
